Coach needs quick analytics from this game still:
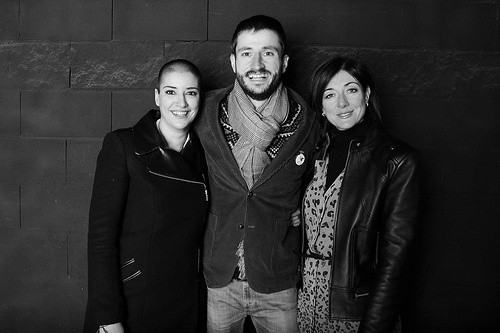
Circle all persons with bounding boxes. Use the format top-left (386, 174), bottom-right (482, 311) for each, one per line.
top-left (192, 13), bottom-right (323, 333)
top-left (81, 58), bottom-right (301, 333)
top-left (290, 56), bottom-right (423, 333)
